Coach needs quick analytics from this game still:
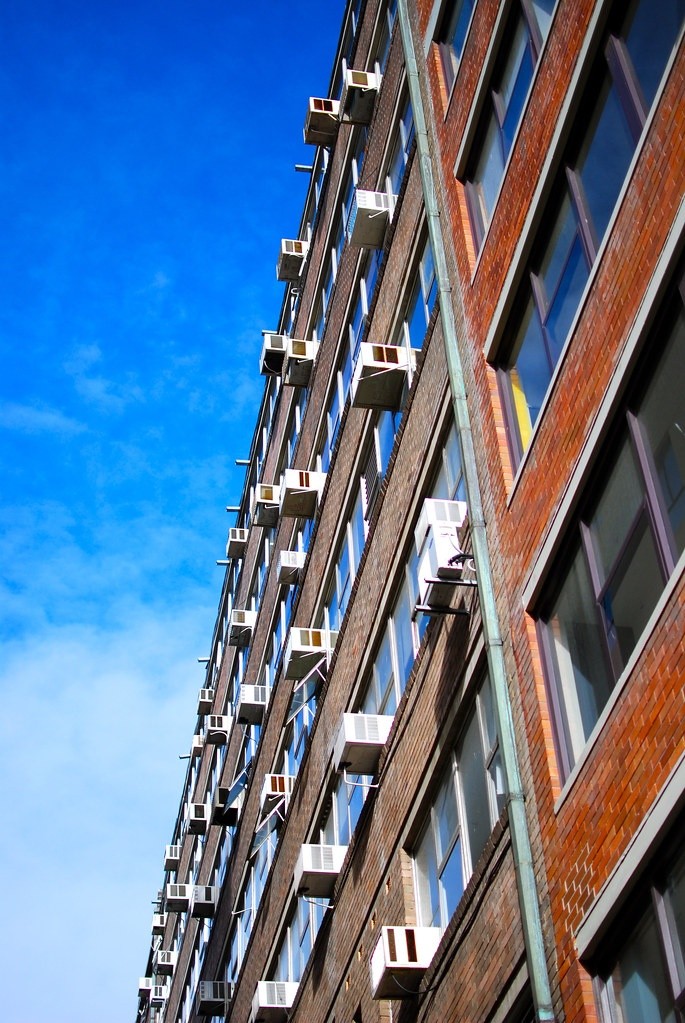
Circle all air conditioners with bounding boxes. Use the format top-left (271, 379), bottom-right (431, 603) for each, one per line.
top-left (204, 714), bottom-right (232, 746)
top-left (414, 497), bottom-right (467, 617)
top-left (281, 339), bottom-right (320, 387)
top-left (343, 69), bottom-right (383, 124)
top-left (252, 482), bottom-right (280, 528)
top-left (155, 950), bottom-right (173, 975)
top-left (137, 977), bottom-right (151, 996)
top-left (251, 981), bottom-right (299, 1023)
top-left (150, 985), bottom-right (166, 1007)
top-left (195, 981), bottom-right (225, 1015)
top-left (163, 845), bottom-right (179, 871)
top-left (228, 609), bottom-right (257, 647)
top-left (279, 468), bottom-right (320, 519)
top-left (276, 550), bottom-right (301, 584)
top-left (275, 239), bottom-right (308, 282)
top-left (347, 188), bottom-right (398, 249)
top-left (350, 341), bottom-right (407, 411)
top-left (151, 914), bottom-right (164, 935)
top-left (333, 713), bottom-right (394, 776)
top-left (192, 735), bottom-right (204, 756)
top-left (210, 786), bottom-right (239, 826)
top-left (368, 925), bottom-right (442, 1000)
top-left (164, 884), bottom-right (190, 912)
top-left (190, 885), bottom-right (215, 919)
top-left (295, 844), bottom-right (348, 898)
top-left (235, 684), bottom-right (266, 726)
top-left (259, 333), bottom-right (286, 376)
top-left (304, 96), bottom-right (340, 146)
top-left (185, 803), bottom-right (208, 835)
top-left (226, 528), bottom-right (249, 559)
top-left (259, 774), bottom-right (285, 814)
top-left (196, 688), bottom-right (214, 715)
